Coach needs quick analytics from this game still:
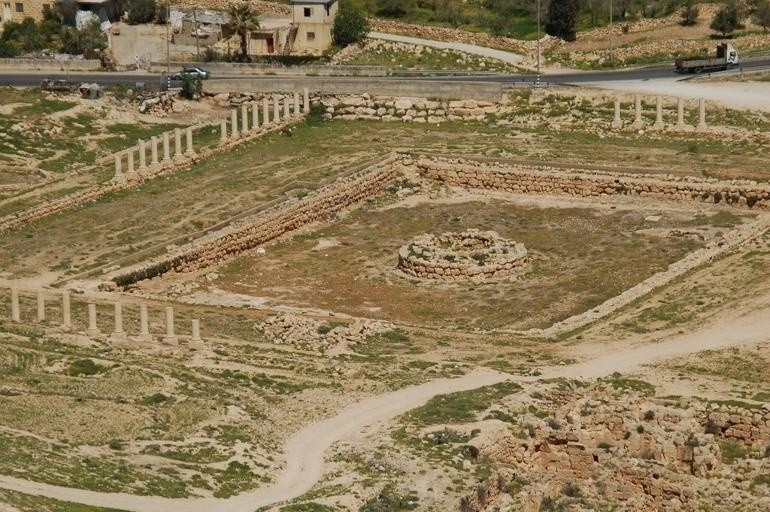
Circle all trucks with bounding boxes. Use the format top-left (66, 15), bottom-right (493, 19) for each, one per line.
top-left (675, 42), bottom-right (740, 73)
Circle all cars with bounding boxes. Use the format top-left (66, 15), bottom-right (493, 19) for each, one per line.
top-left (170, 67), bottom-right (209, 81)
